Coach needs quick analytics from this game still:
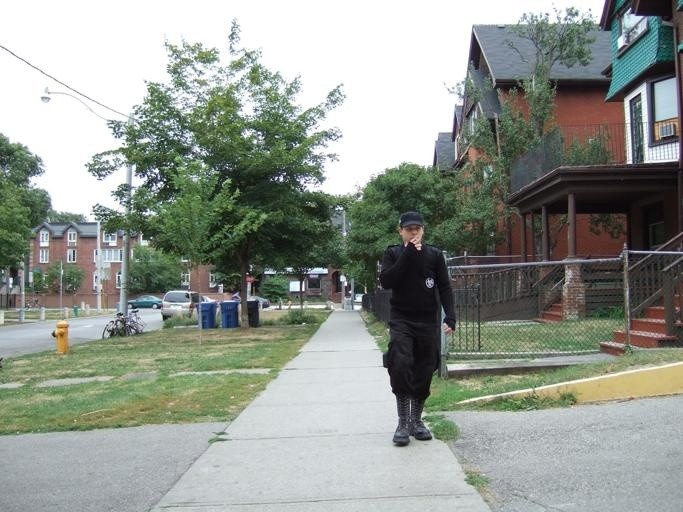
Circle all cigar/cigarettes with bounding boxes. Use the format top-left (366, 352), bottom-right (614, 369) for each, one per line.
top-left (414, 238), bottom-right (417, 241)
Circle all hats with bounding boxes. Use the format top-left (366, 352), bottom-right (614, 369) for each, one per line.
top-left (398, 212), bottom-right (425, 227)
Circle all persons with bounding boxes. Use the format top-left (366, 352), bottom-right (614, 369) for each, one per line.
top-left (381, 210), bottom-right (456, 448)
top-left (190, 288), bottom-right (204, 314)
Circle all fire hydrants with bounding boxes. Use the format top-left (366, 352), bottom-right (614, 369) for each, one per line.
top-left (73, 306), bottom-right (78, 317)
top-left (52, 319), bottom-right (69, 354)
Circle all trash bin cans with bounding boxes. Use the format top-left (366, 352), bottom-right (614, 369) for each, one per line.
top-left (343, 296), bottom-right (353, 311)
top-left (196, 302), bottom-right (218, 328)
top-left (247, 300), bottom-right (261, 327)
top-left (220, 301), bottom-right (241, 328)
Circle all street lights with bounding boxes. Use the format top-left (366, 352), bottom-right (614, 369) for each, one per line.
top-left (340, 273), bottom-right (347, 308)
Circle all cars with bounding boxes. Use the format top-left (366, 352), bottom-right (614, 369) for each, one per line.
top-left (161, 291), bottom-right (219, 320)
top-left (354, 294), bottom-right (365, 302)
top-left (247, 296), bottom-right (270, 308)
top-left (128, 295), bottom-right (162, 308)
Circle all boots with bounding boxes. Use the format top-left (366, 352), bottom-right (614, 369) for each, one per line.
top-left (409, 398), bottom-right (433, 441)
top-left (391, 391), bottom-right (410, 445)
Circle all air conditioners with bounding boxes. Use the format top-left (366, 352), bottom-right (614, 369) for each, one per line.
top-left (659, 124), bottom-right (676, 138)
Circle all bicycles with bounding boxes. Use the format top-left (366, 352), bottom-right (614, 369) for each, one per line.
top-left (26, 298), bottom-right (41, 310)
top-left (103, 310), bottom-right (145, 339)
